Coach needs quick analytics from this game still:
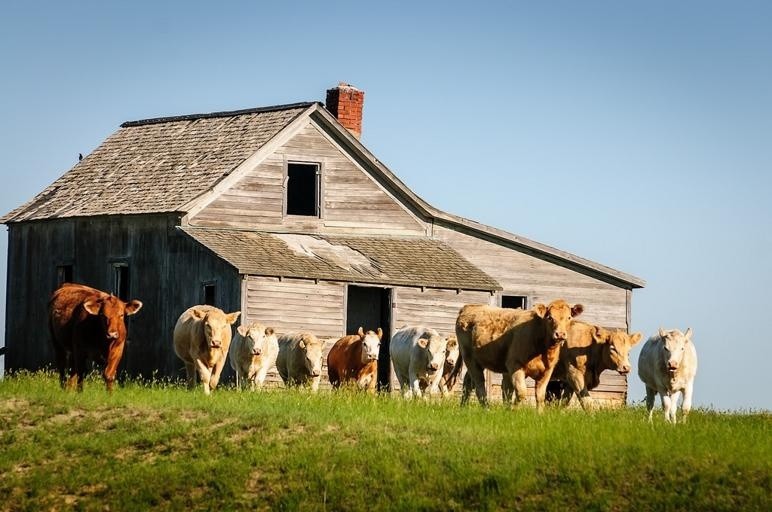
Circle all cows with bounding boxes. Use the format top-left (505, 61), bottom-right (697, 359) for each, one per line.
top-left (326, 324), bottom-right (384, 394)
top-left (636, 324), bottom-right (699, 427)
top-left (44, 281), bottom-right (143, 397)
top-left (172, 299), bottom-right (242, 395)
top-left (232, 317), bottom-right (278, 393)
top-left (277, 328), bottom-right (327, 395)
top-left (388, 298), bottom-right (643, 426)
